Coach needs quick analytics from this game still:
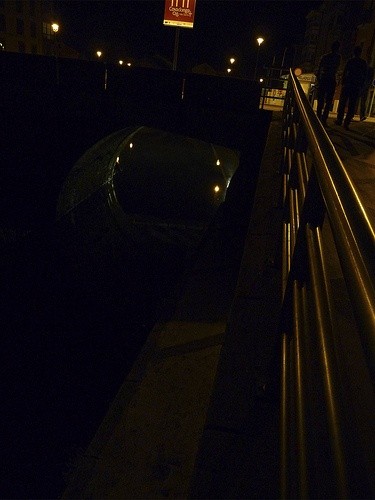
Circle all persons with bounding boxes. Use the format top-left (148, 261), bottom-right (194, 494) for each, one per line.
top-left (315, 42), bottom-right (341, 129)
top-left (349, 55), bottom-right (375, 121)
top-left (308, 77), bottom-right (315, 107)
top-left (333, 46), bottom-right (367, 127)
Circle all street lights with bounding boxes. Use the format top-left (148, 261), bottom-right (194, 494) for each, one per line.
top-left (50, 19), bottom-right (61, 84)
top-left (253, 34), bottom-right (265, 74)
